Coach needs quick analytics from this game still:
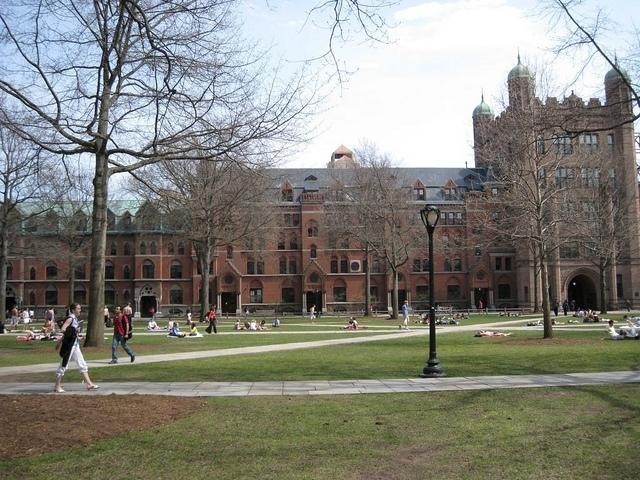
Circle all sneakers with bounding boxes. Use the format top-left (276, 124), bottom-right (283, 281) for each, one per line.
top-left (87, 384), bottom-right (100, 391)
top-left (54, 386), bottom-right (65, 393)
top-left (108, 359), bottom-right (117, 364)
top-left (131, 354), bottom-right (135, 362)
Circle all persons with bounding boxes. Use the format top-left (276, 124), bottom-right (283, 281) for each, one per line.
top-left (203, 308), bottom-right (218, 334)
top-left (122, 301), bottom-right (133, 315)
top-left (607, 314), bottom-right (640, 340)
top-left (414, 312), bottom-right (471, 326)
top-left (479, 299), bottom-right (484, 314)
top-left (397, 299), bottom-right (410, 330)
top-left (53, 302), bottom-right (101, 393)
top-left (272, 317), bottom-right (280, 327)
top-left (309, 303), bottom-right (316, 322)
top-left (475, 329), bottom-right (512, 337)
top-left (107, 304), bottom-right (136, 364)
top-left (105, 318), bottom-right (113, 328)
top-left (244, 305), bottom-right (250, 318)
top-left (104, 304), bottom-right (110, 322)
top-left (348, 316), bottom-right (354, 325)
top-left (146, 310), bottom-right (199, 338)
top-left (234, 318), bottom-right (268, 331)
top-left (624, 299), bottom-right (634, 312)
top-left (527, 300), bottom-right (610, 327)
top-left (342, 318), bottom-right (359, 330)
top-left (0, 305), bottom-right (85, 342)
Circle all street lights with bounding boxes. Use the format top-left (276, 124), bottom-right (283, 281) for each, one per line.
top-left (419, 204), bottom-right (444, 373)
